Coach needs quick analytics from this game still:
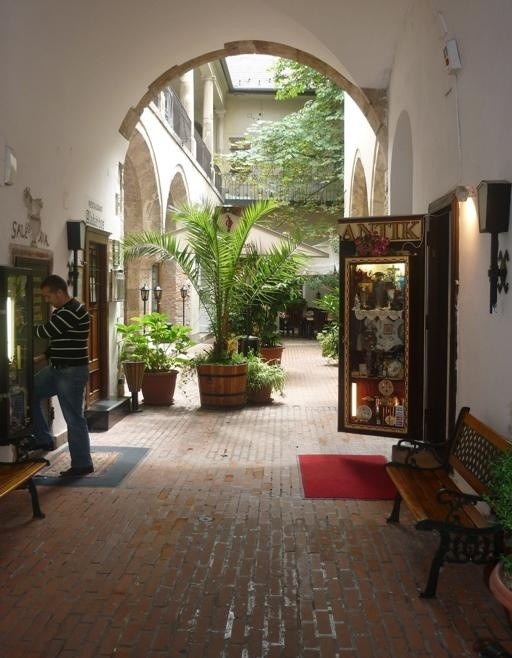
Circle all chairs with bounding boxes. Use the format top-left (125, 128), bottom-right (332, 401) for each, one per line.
top-left (280, 297), bottom-right (335, 340)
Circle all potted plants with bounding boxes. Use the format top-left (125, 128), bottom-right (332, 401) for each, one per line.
top-left (117, 195), bottom-right (307, 414)
top-left (482, 417), bottom-right (512, 632)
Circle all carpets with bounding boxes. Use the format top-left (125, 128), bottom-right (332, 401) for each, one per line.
top-left (34, 451), bottom-right (119, 478)
top-left (298, 454), bottom-right (401, 501)
top-left (29, 446), bottom-right (150, 487)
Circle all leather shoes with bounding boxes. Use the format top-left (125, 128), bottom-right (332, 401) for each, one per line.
top-left (60, 466), bottom-right (94, 476)
top-left (26, 438), bottom-right (54, 450)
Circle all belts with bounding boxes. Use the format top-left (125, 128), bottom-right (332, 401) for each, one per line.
top-left (51, 362), bottom-right (78, 368)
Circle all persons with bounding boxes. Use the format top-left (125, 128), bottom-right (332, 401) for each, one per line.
top-left (15, 273), bottom-right (94, 477)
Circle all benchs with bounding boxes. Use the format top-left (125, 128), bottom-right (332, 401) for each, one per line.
top-left (385, 406), bottom-right (512, 598)
top-left (0, 458), bottom-right (50, 518)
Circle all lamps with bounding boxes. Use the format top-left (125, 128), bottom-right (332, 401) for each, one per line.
top-left (476, 181), bottom-right (511, 313)
top-left (66, 220), bottom-right (86, 297)
